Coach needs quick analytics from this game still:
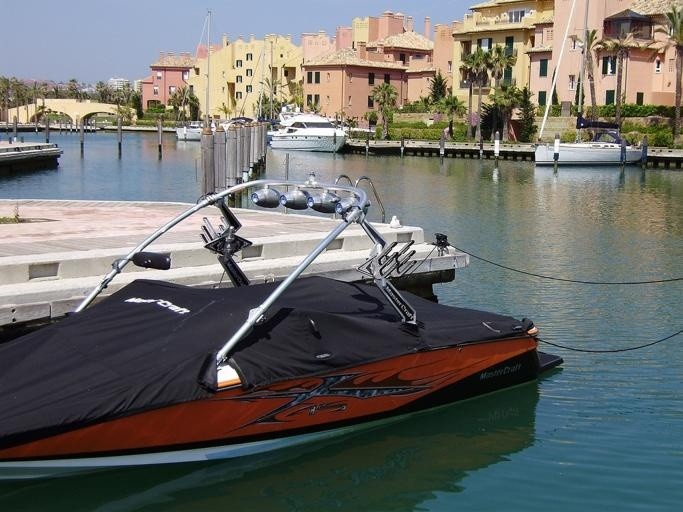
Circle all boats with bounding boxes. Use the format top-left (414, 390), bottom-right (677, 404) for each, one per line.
top-left (1, 172), bottom-right (564, 480)
top-left (269, 103), bottom-right (349, 153)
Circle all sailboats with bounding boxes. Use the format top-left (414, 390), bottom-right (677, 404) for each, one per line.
top-left (174, 10), bottom-right (272, 147)
top-left (533, 0), bottom-right (644, 167)
